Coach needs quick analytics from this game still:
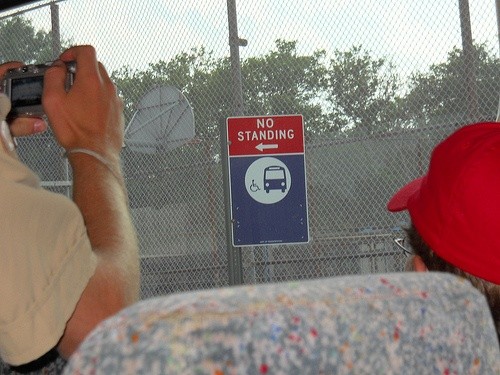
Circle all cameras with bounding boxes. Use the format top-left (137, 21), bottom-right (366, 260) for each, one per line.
top-left (3, 59), bottom-right (78, 117)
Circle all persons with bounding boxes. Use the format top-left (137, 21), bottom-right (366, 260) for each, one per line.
top-left (387, 122), bottom-right (500, 350)
top-left (0, 45), bottom-right (140, 375)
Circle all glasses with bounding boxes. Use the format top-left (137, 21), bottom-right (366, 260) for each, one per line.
top-left (392, 237), bottom-right (432, 271)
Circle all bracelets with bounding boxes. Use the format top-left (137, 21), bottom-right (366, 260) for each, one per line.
top-left (64, 148), bottom-right (127, 195)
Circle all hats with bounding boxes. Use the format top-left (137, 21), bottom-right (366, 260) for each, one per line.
top-left (386, 121), bottom-right (500, 285)
